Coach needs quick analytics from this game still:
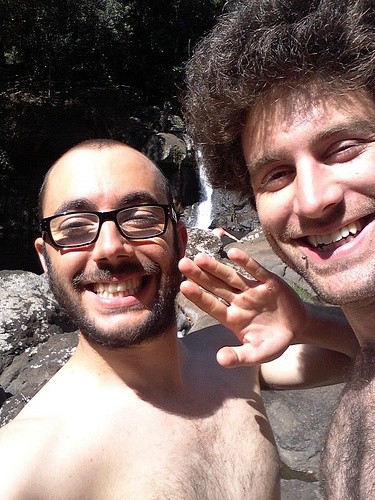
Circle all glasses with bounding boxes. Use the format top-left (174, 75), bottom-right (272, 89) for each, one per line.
top-left (39, 203), bottom-right (171, 248)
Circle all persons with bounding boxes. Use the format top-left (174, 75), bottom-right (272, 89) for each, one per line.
top-left (0, 138), bottom-right (360, 500)
top-left (182, 0), bottom-right (375, 500)
